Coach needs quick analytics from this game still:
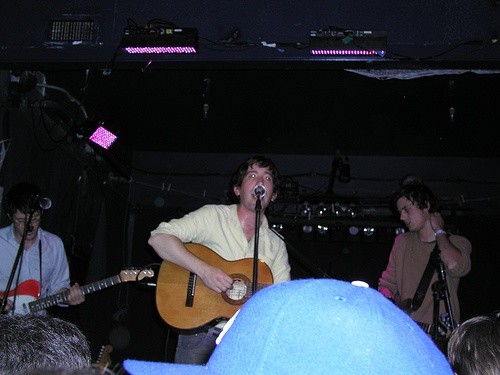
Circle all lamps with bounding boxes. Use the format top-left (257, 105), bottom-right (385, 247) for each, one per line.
top-left (337, 159), bottom-right (351, 183)
top-left (119, 28), bottom-right (199, 53)
top-left (310, 29), bottom-right (388, 54)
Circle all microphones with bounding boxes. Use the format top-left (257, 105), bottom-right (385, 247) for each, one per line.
top-left (253, 185), bottom-right (266, 199)
top-left (30, 194), bottom-right (52, 209)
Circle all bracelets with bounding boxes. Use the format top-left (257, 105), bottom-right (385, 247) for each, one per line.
top-left (434, 230), bottom-right (447, 236)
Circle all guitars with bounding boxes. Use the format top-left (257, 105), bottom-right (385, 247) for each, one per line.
top-left (379, 287), bottom-right (448, 338)
top-left (155, 242), bottom-right (274, 336)
top-left (0, 266), bottom-right (154, 316)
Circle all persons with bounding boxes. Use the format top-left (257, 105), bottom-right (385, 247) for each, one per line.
top-left (447, 316), bottom-right (500, 375)
top-left (0, 191), bottom-right (85, 317)
top-left (148, 156), bottom-right (292, 366)
top-left (0, 313), bottom-right (92, 375)
top-left (378, 186), bottom-right (471, 358)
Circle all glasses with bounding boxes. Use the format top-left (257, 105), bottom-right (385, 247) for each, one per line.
top-left (10, 212), bottom-right (41, 224)
